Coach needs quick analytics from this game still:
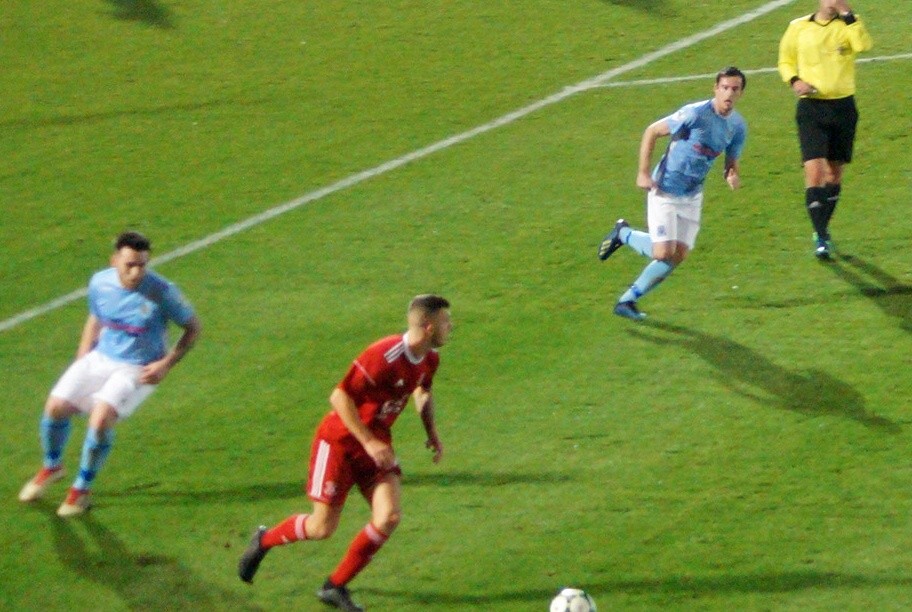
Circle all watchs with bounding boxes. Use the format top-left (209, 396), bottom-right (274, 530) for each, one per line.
top-left (844, 10), bottom-right (852, 16)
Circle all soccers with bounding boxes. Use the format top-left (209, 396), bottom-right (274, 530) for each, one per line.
top-left (549, 587), bottom-right (597, 612)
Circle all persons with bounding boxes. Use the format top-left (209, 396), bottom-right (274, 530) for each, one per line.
top-left (597, 67), bottom-right (749, 322)
top-left (238, 294), bottom-right (454, 612)
top-left (19, 232), bottom-right (202, 517)
top-left (777, 0), bottom-right (875, 259)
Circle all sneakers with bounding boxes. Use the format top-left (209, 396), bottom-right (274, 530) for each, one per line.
top-left (237, 528), bottom-right (270, 582)
top-left (598, 219), bottom-right (629, 260)
top-left (18, 465), bottom-right (64, 501)
top-left (613, 301), bottom-right (646, 320)
top-left (815, 242), bottom-right (830, 256)
top-left (315, 580), bottom-right (363, 612)
top-left (56, 487), bottom-right (91, 517)
